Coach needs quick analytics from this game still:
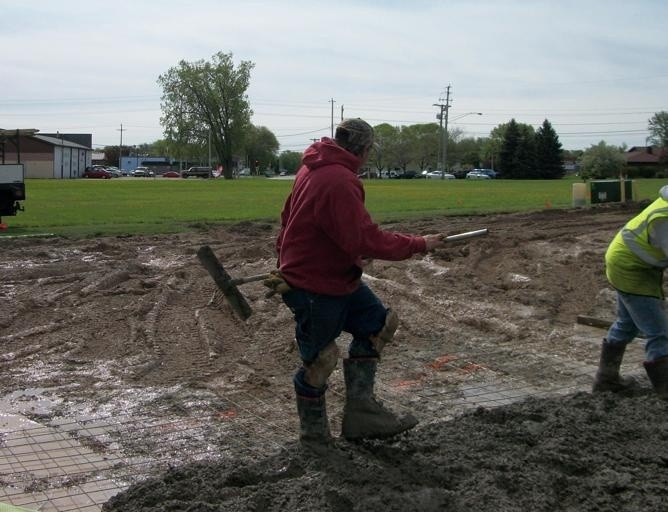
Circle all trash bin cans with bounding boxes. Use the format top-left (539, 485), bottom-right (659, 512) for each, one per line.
top-left (625, 181), bottom-right (632, 201)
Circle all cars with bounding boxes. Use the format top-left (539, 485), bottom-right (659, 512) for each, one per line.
top-left (83, 165), bottom-right (155, 179)
top-left (357, 170), bottom-right (399, 180)
top-left (163, 172), bottom-right (180, 177)
top-left (426, 170), bottom-right (456, 179)
top-left (466, 168), bottom-right (496, 179)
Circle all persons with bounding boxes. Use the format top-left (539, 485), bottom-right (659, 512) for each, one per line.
top-left (592, 178), bottom-right (668, 398)
top-left (262, 116), bottom-right (449, 446)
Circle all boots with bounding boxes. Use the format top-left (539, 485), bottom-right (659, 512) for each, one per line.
top-left (593, 338), bottom-right (634, 389)
top-left (342, 358), bottom-right (419, 440)
top-left (297, 395), bottom-right (330, 445)
top-left (642, 355), bottom-right (668, 398)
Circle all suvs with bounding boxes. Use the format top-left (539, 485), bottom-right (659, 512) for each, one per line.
top-left (182, 167), bottom-right (213, 178)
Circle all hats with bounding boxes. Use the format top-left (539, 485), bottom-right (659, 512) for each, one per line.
top-left (336, 117), bottom-right (379, 151)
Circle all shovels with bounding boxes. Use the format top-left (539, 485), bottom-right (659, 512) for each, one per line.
top-left (197, 227), bottom-right (490, 320)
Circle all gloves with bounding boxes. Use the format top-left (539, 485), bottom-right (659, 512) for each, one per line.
top-left (263, 270), bottom-right (290, 299)
